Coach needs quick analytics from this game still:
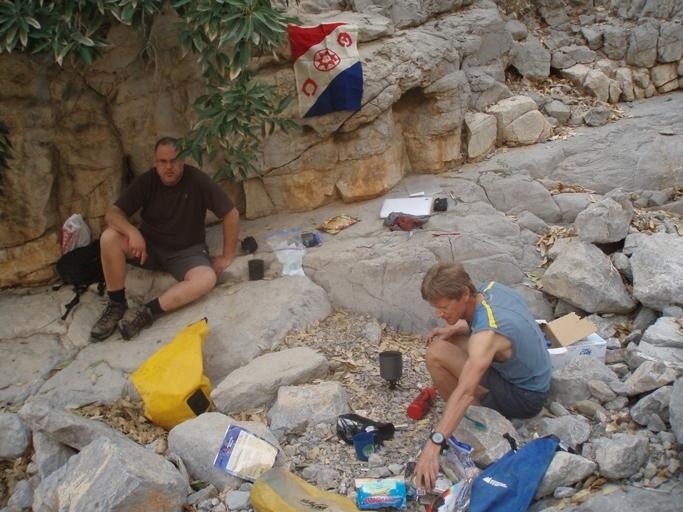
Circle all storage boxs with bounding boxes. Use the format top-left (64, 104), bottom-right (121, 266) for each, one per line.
top-left (533, 310), bottom-right (608, 376)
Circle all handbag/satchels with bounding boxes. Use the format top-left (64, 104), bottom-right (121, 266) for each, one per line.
top-left (56, 239), bottom-right (103, 286)
top-left (466, 435), bottom-right (560, 512)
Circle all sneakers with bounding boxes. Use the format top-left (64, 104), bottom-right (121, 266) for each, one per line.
top-left (117, 306), bottom-right (154, 340)
top-left (91, 299), bottom-right (128, 340)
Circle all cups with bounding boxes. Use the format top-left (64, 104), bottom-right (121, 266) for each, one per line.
top-left (352, 431), bottom-right (375, 461)
top-left (248, 258), bottom-right (264, 280)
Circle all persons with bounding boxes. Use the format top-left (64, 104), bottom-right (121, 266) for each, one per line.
top-left (412, 260), bottom-right (552, 497)
top-left (90, 136), bottom-right (240, 341)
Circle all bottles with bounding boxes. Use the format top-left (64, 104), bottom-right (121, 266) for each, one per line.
top-left (408, 384), bottom-right (438, 420)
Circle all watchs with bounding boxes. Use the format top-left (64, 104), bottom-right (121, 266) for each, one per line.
top-left (428, 431), bottom-right (450, 456)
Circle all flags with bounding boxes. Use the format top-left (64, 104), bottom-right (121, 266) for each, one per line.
top-left (287, 23), bottom-right (363, 118)
